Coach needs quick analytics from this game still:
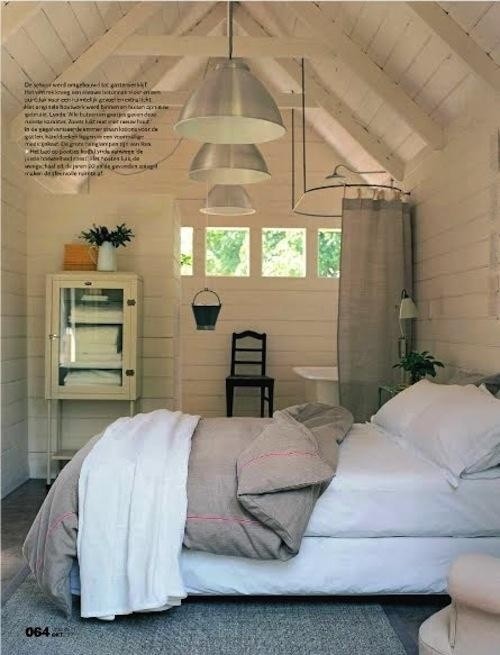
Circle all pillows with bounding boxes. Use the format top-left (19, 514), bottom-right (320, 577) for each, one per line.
top-left (365, 368), bottom-right (500, 492)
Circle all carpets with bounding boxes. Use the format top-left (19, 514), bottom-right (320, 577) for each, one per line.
top-left (1, 565), bottom-right (417, 655)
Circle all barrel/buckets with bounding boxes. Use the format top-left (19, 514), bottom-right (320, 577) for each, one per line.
top-left (191, 288), bottom-right (223, 331)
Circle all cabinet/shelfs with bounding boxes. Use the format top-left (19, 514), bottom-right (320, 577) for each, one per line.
top-left (45, 272), bottom-right (146, 499)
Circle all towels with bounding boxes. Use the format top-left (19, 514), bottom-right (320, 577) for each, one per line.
top-left (61, 305), bottom-right (123, 385)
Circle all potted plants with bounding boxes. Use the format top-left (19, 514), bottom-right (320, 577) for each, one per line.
top-left (77, 219), bottom-right (138, 270)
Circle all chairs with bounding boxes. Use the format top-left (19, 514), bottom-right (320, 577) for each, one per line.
top-left (417, 548), bottom-right (500, 655)
top-left (226, 330), bottom-right (275, 418)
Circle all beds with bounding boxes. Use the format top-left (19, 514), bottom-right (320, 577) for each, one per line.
top-left (23, 374), bottom-right (500, 625)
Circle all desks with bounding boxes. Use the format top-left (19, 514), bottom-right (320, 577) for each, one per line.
top-left (378, 383), bottom-right (407, 409)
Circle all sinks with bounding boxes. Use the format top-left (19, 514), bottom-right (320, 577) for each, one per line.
top-left (290, 365), bottom-right (340, 408)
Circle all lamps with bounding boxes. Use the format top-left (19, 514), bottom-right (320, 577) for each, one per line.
top-left (395, 286), bottom-right (420, 358)
top-left (186, 144), bottom-right (273, 186)
top-left (172, 3), bottom-right (287, 146)
top-left (197, 185), bottom-right (258, 218)
